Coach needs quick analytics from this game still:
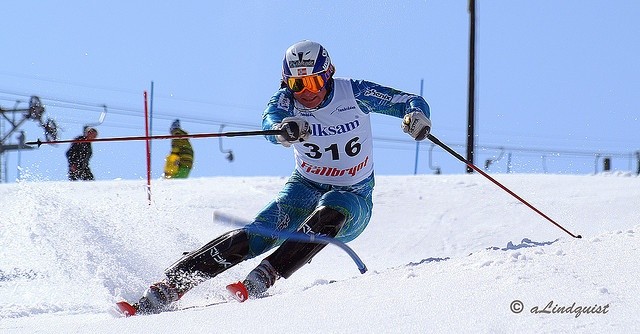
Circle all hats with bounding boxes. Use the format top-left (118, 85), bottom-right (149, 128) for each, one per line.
top-left (84, 126), bottom-right (98, 138)
top-left (170, 119), bottom-right (180, 132)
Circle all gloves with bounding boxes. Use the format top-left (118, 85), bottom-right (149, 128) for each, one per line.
top-left (401, 111), bottom-right (431, 141)
top-left (273, 117), bottom-right (312, 147)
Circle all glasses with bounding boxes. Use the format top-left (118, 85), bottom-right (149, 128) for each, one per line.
top-left (282, 64), bottom-right (334, 94)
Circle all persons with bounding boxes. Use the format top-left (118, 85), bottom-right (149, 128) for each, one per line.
top-left (116, 39), bottom-right (432, 317)
top-left (63, 127), bottom-right (103, 180)
top-left (163, 119), bottom-right (194, 177)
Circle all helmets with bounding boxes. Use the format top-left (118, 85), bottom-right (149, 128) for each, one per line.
top-left (281, 40), bottom-right (330, 78)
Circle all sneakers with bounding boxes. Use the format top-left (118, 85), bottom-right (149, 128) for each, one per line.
top-left (139, 277), bottom-right (184, 314)
top-left (244, 260), bottom-right (281, 296)
